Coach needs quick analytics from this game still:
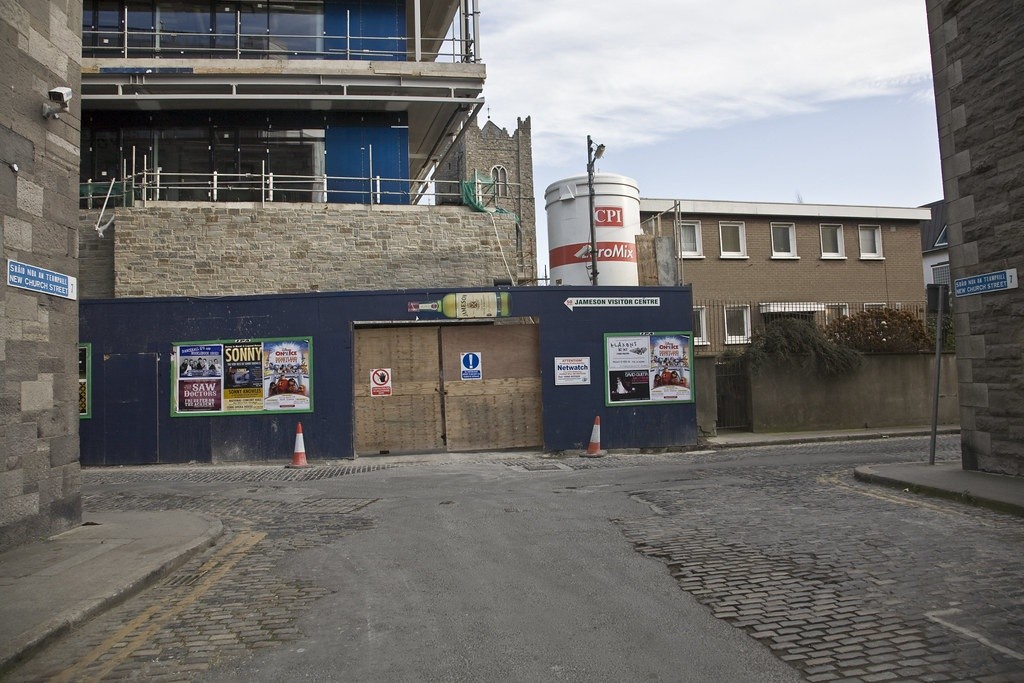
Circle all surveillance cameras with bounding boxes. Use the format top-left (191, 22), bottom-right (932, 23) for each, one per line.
top-left (574, 245), bottom-right (592, 258)
top-left (49, 87), bottom-right (73, 103)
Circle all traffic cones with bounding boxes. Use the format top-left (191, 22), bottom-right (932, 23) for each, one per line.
top-left (284, 422), bottom-right (314, 470)
top-left (578, 416), bottom-right (608, 460)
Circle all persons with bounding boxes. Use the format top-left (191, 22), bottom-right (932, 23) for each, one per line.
top-left (180, 358), bottom-right (221, 378)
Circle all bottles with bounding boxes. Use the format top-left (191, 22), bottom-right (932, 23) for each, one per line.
top-left (408, 292), bottom-right (513, 317)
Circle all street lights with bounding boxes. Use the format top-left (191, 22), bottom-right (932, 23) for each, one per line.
top-left (585, 135), bottom-right (608, 288)
